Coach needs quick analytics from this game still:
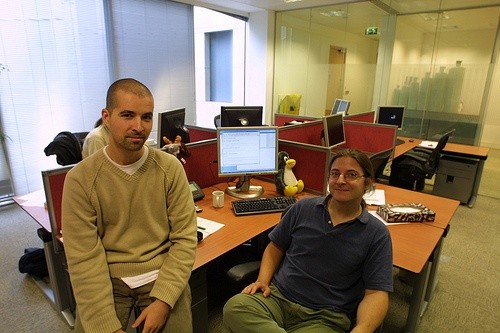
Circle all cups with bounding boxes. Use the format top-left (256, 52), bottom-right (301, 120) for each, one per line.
top-left (212, 190), bottom-right (224, 208)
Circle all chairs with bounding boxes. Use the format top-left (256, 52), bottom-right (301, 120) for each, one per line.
top-left (392, 128), bottom-right (455, 190)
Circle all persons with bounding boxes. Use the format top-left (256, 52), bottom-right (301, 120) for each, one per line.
top-left (82, 117), bottom-right (109, 159)
top-left (61, 78), bottom-right (197, 333)
top-left (223, 148), bottom-right (392, 333)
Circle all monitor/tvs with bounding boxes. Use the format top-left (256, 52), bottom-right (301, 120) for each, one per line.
top-left (321, 98), bottom-right (351, 148)
top-left (216, 126), bottom-right (278, 199)
top-left (376, 106), bottom-right (405, 130)
top-left (157, 108), bottom-right (186, 149)
top-left (220, 106), bottom-right (263, 126)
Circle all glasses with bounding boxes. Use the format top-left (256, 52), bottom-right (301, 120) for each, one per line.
top-left (329, 170), bottom-right (364, 181)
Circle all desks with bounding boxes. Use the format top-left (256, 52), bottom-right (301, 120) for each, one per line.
top-left (394, 137), bottom-right (488, 207)
top-left (190, 175), bottom-right (461, 333)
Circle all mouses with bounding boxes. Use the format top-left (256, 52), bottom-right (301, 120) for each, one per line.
top-left (409, 139), bottom-right (414, 142)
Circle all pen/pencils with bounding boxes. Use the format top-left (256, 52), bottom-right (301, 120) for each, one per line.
top-left (197, 226), bottom-right (205, 230)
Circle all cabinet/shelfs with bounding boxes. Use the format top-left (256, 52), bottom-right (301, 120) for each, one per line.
top-left (433, 156), bottom-right (479, 204)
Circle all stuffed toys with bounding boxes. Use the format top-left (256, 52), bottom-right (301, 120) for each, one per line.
top-left (274, 152), bottom-right (305, 197)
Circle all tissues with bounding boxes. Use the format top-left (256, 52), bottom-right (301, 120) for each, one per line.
top-left (376, 202), bottom-right (436, 223)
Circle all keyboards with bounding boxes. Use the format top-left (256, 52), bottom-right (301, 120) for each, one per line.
top-left (232, 196), bottom-right (298, 215)
top-left (396, 139), bottom-right (405, 145)
